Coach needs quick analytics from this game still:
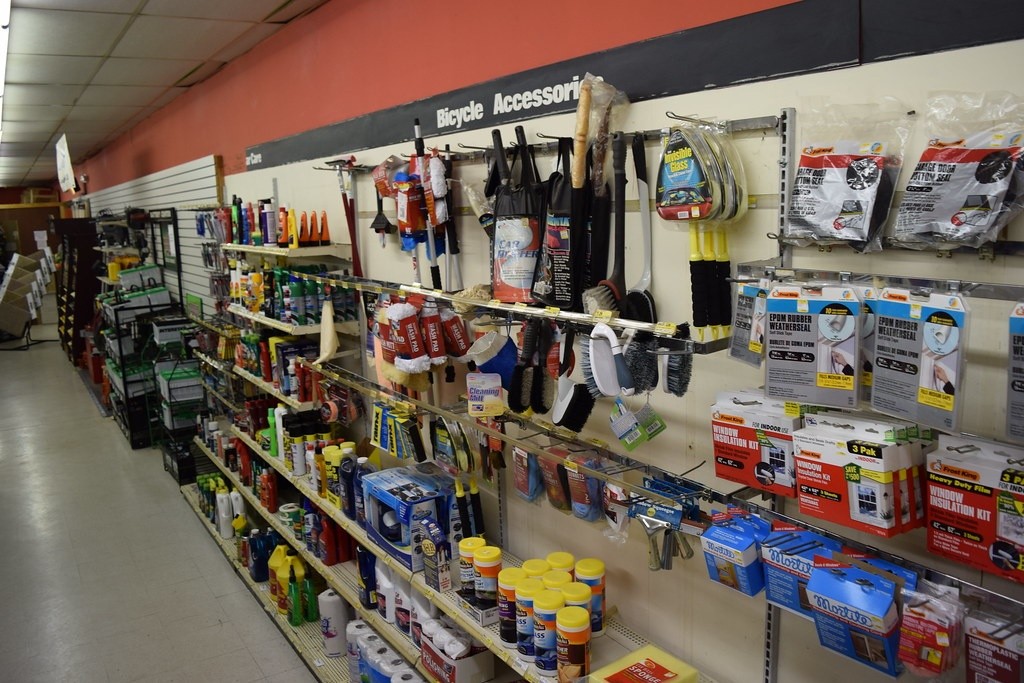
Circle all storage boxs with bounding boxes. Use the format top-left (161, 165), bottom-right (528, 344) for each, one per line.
top-left (82, 265), bottom-right (205, 431)
top-left (699, 388), bottom-right (1024, 683)
top-left (268, 335), bottom-right (328, 403)
top-left (360, 463), bottom-right (499, 683)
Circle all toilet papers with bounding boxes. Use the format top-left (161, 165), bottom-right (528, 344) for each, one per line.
top-left (318, 588), bottom-right (425, 683)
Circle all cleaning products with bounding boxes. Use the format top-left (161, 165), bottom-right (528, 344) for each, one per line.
top-left (357, 546), bottom-right (429, 652)
top-left (197, 194), bottom-right (372, 626)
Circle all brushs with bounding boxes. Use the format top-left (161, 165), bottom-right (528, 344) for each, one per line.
top-left (370, 72), bottom-right (748, 573)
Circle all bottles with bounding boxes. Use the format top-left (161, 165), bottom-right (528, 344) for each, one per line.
top-left (196, 194), bottom-right (442, 651)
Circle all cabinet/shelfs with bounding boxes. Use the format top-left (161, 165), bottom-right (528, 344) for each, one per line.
top-left (99, 272), bottom-right (185, 450)
top-left (179, 240), bottom-right (763, 683)
top-left (48, 217), bottom-right (103, 368)
top-left (142, 333), bottom-right (212, 486)
